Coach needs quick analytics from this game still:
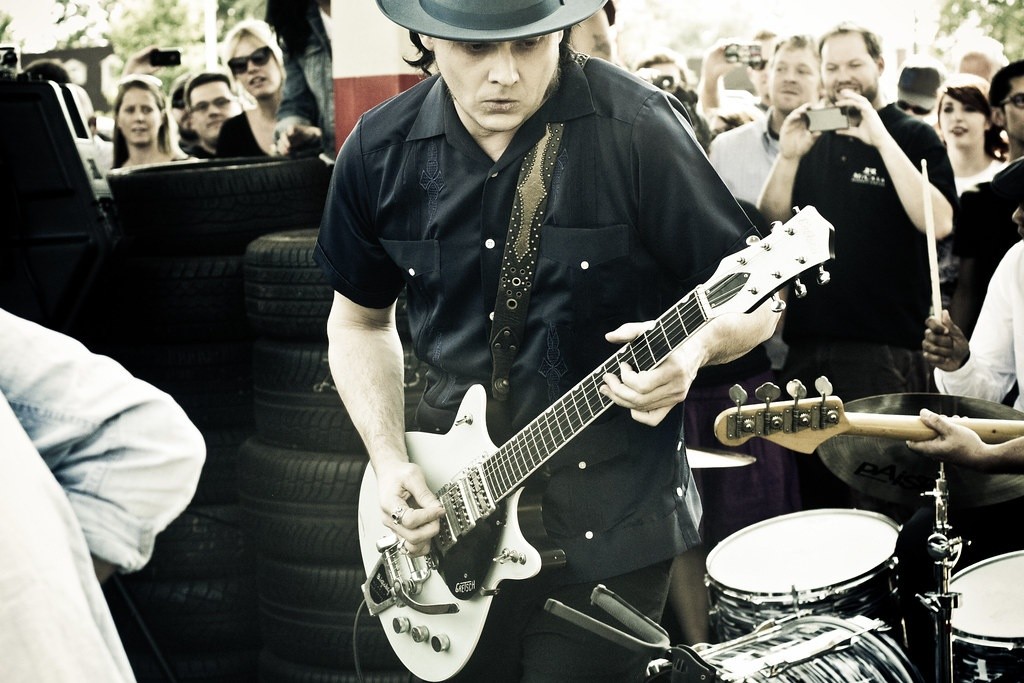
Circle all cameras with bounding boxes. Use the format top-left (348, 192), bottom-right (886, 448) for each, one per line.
top-left (652, 76), bottom-right (674, 89)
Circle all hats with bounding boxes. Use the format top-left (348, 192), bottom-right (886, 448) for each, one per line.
top-left (380, 2), bottom-right (609, 40)
top-left (899, 67), bottom-right (940, 97)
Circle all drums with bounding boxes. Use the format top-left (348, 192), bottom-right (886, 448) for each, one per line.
top-left (641, 614), bottom-right (923, 683)
top-left (701, 505), bottom-right (901, 645)
top-left (935, 550), bottom-right (1023, 682)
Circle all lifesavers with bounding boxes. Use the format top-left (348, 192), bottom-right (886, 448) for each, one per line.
top-left (105, 154), bottom-right (413, 683)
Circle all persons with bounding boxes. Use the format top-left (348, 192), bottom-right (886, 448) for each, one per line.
top-left (111, 73), bottom-right (177, 167)
top-left (756, 20), bottom-right (959, 508)
top-left (125, 45), bottom-right (243, 159)
top-left (905, 408), bottom-right (1024, 475)
top-left (265, 0), bottom-right (335, 157)
top-left (214, 18), bottom-right (286, 155)
top-left (709, 35), bottom-right (820, 209)
top-left (637, 30), bottom-right (778, 153)
top-left (894, 37), bottom-right (1024, 339)
top-left (922, 160), bottom-right (1024, 419)
top-left (0, 308), bottom-right (207, 583)
top-left (310, 0), bottom-right (781, 683)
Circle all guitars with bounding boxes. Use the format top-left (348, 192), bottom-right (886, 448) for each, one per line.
top-left (710, 376), bottom-right (1024, 460)
top-left (356, 200), bottom-right (838, 683)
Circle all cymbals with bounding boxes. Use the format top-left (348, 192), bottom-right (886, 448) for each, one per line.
top-left (815, 389), bottom-right (1024, 510)
top-left (684, 442), bottom-right (757, 471)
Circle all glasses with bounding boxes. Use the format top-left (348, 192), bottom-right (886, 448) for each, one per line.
top-left (228, 46), bottom-right (273, 75)
top-left (999, 93), bottom-right (1024, 109)
top-left (897, 100), bottom-right (932, 116)
top-left (188, 96), bottom-right (230, 115)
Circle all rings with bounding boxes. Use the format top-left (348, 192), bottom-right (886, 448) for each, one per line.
top-left (397, 538), bottom-right (410, 555)
top-left (392, 504), bottom-right (407, 525)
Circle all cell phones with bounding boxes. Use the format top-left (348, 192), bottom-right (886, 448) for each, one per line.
top-left (150, 50), bottom-right (182, 66)
top-left (805, 106), bottom-right (851, 132)
top-left (724, 44), bottom-right (763, 65)
